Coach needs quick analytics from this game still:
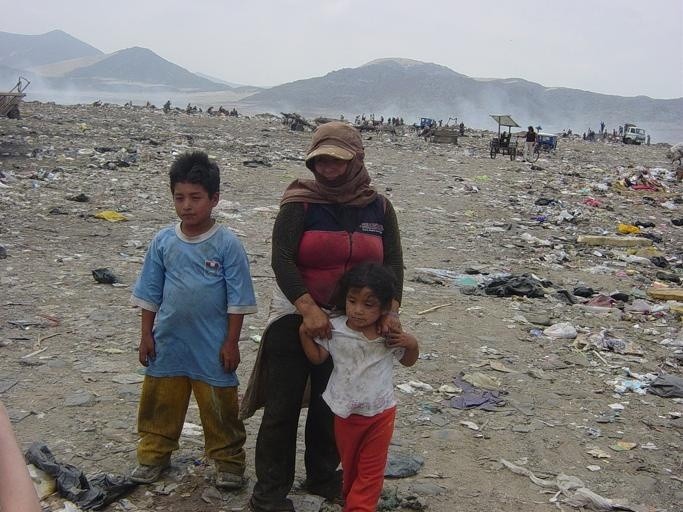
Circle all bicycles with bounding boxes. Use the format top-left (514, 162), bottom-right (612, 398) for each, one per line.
top-left (509, 135), bottom-right (539, 163)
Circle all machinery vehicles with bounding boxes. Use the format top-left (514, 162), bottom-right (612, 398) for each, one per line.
top-left (534, 126), bottom-right (558, 152)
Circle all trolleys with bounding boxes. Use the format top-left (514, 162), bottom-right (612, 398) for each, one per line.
top-left (0, 76), bottom-right (32, 119)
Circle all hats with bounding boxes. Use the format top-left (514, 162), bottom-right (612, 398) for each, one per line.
top-left (304, 121), bottom-right (367, 161)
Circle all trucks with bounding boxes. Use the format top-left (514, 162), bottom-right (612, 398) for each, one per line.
top-left (608, 127), bottom-right (645, 145)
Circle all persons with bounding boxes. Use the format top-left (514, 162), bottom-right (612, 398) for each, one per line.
top-left (340, 111), bottom-right (344, 120)
top-left (355, 112), bottom-right (405, 127)
top-left (500, 125), bottom-right (542, 163)
top-left (244, 121), bottom-right (402, 511)
top-left (562, 120), bottom-right (624, 143)
top-left (128, 152), bottom-right (258, 491)
top-left (417, 119), bottom-right (465, 143)
top-left (647, 134), bottom-right (651, 145)
top-left (146, 100), bottom-right (239, 119)
top-left (299, 260), bottom-right (419, 511)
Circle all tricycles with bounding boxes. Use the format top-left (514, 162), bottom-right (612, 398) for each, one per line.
top-left (489, 113), bottom-right (520, 162)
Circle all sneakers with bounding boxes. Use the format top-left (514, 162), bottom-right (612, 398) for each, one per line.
top-left (308, 470), bottom-right (343, 490)
top-left (217, 472), bottom-right (241, 486)
top-left (130, 464), bottom-right (170, 483)
